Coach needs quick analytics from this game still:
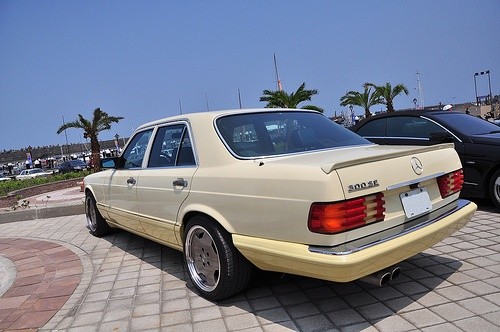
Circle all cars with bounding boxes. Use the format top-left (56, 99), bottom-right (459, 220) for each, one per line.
top-left (59, 159), bottom-right (92, 175)
top-left (83, 109), bottom-right (478, 302)
top-left (87, 158), bottom-right (101, 169)
top-left (16, 167), bottom-right (53, 179)
top-left (352, 109), bottom-right (500, 209)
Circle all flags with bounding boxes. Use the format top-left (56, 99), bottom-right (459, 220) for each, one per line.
top-left (26, 152), bottom-right (32, 166)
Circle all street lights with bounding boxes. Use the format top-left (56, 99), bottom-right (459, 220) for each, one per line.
top-left (115, 133), bottom-right (120, 152)
top-left (350, 106), bottom-right (355, 123)
top-left (413, 98), bottom-right (417, 109)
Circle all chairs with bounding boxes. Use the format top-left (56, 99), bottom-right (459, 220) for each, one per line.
top-left (290, 127), bottom-right (326, 150)
top-left (171, 146), bottom-right (195, 165)
top-left (399, 120), bottom-right (421, 137)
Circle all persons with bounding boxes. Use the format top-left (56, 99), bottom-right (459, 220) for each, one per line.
top-left (465, 107), bottom-right (470, 115)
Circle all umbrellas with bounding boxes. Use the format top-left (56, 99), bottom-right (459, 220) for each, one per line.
top-left (443, 104), bottom-right (452, 110)
